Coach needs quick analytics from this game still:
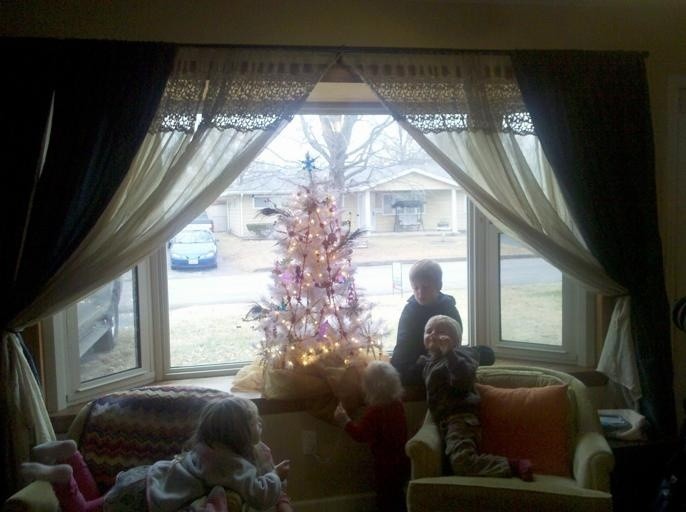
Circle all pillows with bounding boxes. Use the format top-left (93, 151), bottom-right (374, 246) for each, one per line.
top-left (468, 380), bottom-right (572, 477)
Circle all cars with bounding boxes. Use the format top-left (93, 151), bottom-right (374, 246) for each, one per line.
top-left (166, 208), bottom-right (221, 271)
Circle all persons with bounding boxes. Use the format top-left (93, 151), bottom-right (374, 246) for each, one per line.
top-left (416, 314), bottom-right (534, 482)
top-left (333, 360), bottom-right (411, 512)
top-left (390, 258), bottom-right (464, 386)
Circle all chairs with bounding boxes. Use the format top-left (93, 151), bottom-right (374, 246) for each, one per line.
top-left (404, 364), bottom-right (615, 511)
